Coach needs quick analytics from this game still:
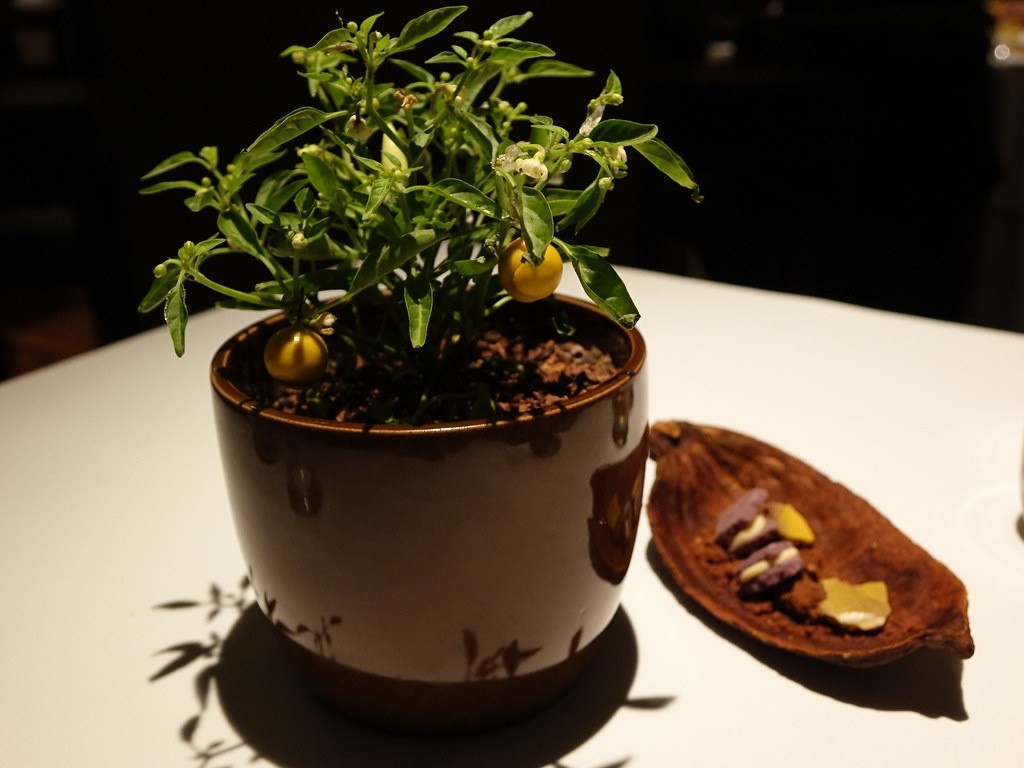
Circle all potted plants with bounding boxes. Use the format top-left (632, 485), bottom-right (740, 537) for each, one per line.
top-left (152, 6), bottom-right (704, 745)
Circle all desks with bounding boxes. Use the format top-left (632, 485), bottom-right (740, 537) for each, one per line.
top-left (0, 239), bottom-right (1024, 768)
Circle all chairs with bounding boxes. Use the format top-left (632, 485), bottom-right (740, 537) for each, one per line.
top-left (637, 0), bottom-right (1010, 325)
top-left (0, 0), bottom-right (139, 382)
